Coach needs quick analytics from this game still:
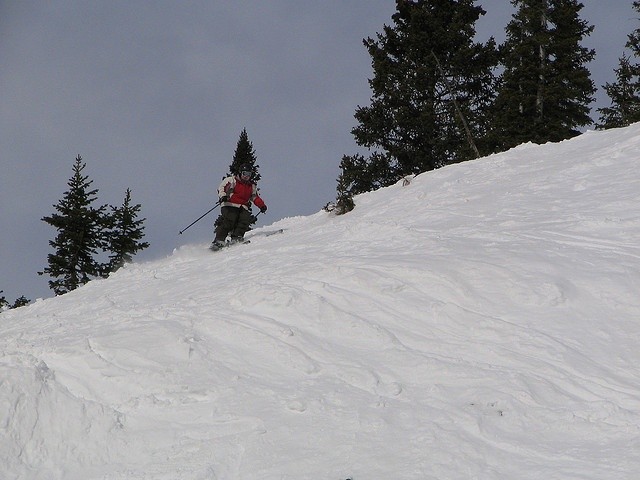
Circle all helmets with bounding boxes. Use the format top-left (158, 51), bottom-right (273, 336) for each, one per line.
top-left (238, 161), bottom-right (255, 182)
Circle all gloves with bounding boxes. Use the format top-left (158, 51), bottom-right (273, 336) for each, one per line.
top-left (221, 196), bottom-right (231, 202)
top-left (260, 205), bottom-right (267, 213)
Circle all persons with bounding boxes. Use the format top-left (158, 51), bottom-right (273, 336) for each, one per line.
top-left (211, 164), bottom-right (267, 250)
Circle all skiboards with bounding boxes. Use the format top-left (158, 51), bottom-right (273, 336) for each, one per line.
top-left (225, 229), bottom-right (283, 248)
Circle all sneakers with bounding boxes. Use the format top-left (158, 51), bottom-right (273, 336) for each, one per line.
top-left (231, 237), bottom-right (245, 245)
top-left (209, 237), bottom-right (229, 249)
